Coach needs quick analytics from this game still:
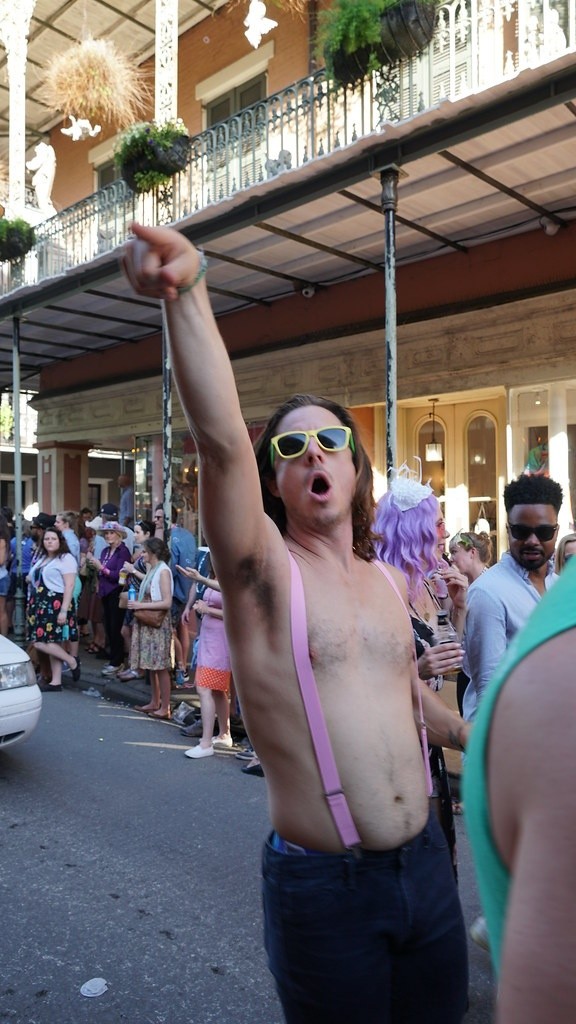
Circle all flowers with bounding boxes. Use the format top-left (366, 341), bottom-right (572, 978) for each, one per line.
top-left (106, 117), bottom-right (190, 193)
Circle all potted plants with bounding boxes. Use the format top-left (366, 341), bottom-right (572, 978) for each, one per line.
top-left (305, 0), bottom-right (440, 101)
top-left (0, 218), bottom-right (37, 263)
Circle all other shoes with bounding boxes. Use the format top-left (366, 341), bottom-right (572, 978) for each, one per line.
top-left (148, 711), bottom-right (171, 720)
top-left (234, 752), bottom-right (256, 760)
top-left (241, 763), bottom-right (266, 778)
top-left (70, 657), bottom-right (81, 681)
top-left (41, 683), bottom-right (62, 692)
top-left (134, 706), bottom-right (160, 712)
top-left (34, 673), bottom-right (52, 685)
top-left (61, 657), bottom-right (77, 673)
top-left (180, 718), bottom-right (204, 736)
top-left (79, 630), bottom-right (190, 687)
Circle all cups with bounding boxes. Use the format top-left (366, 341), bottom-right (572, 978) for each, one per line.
top-left (118, 572), bottom-right (127, 584)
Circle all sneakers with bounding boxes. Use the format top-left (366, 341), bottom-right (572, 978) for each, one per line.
top-left (199, 736), bottom-right (233, 748)
top-left (184, 745), bottom-right (215, 759)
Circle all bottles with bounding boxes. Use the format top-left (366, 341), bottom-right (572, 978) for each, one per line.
top-left (431, 610), bottom-right (461, 675)
top-left (128, 585), bottom-right (136, 602)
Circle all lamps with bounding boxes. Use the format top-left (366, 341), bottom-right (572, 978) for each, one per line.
top-left (425, 399), bottom-right (443, 462)
top-left (469, 420), bottom-right (487, 464)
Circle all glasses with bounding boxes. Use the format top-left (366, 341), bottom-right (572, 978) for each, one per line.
top-left (154, 515), bottom-right (165, 521)
top-left (508, 522), bottom-right (559, 542)
top-left (29, 525), bottom-right (41, 531)
top-left (270, 426), bottom-right (356, 467)
top-left (135, 519), bottom-right (149, 528)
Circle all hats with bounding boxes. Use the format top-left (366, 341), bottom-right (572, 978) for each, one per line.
top-left (31, 512), bottom-right (55, 528)
top-left (97, 503), bottom-right (119, 516)
top-left (95, 521), bottom-right (127, 539)
top-left (85, 516), bottom-right (103, 530)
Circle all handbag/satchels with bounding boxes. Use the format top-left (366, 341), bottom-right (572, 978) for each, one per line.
top-left (133, 588), bottom-right (168, 627)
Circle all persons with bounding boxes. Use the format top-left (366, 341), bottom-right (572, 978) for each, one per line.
top-left (460, 475), bottom-right (564, 722)
top-left (0, 504), bottom-right (264, 777)
top-left (118, 474), bottom-right (134, 531)
top-left (369, 476), bottom-right (468, 883)
top-left (450, 528), bottom-right (491, 585)
top-left (459, 552), bottom-right (576, 1024)
top-left (121, 221), bottom-right (473, 1024)
top-left (555, 533), bottom-right (576, 574)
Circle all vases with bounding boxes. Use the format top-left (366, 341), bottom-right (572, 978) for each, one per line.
top-left (120, 133), bottom-right (189, 194)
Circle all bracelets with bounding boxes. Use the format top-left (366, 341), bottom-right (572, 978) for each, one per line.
top-left (177, 252), bottom-right (207, 294)
top-left (132, 570), bottom-right (136, 574)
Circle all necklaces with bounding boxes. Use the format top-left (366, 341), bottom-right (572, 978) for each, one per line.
top-left (418, 598), bottom-right (431, 621)
top-left (196, 550), bottom-right (209, 593)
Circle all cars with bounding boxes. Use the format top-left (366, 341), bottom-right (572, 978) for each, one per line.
top-left (0, 631), bottom-right (43, 753)
